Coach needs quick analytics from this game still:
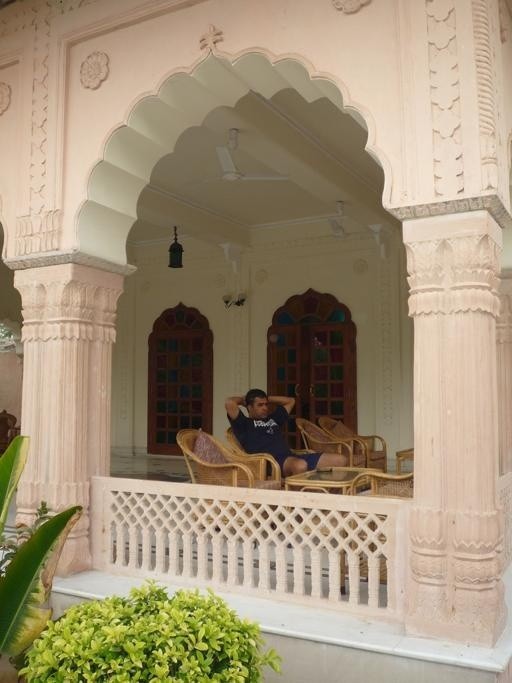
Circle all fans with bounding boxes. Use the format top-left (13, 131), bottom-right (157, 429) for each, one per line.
top-left (201, 128), bottom-right (290, 184)
top-left (326, 201), bottom-right (347, 240)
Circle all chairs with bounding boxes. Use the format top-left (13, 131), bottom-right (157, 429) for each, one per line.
top-left (318, 415), bottom-right (387, 473)
top-left (226, 427), bottom-right (316, 488)
top-left (295, 417), bottom-right (366, 467)
top-left (176, 428), bottom-right (281, 489)
top-left (0, 409), bottom-right (17, 454)
top-left (350, 472), bottom-right (414, 498)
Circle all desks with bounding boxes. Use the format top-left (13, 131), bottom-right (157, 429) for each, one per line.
top-left (396, 448), bottom-right (414, 474)
top-left (284, 467), bottom-right (383, 495)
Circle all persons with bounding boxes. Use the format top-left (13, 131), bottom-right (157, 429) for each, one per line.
top-left (223, 387), bottom-right (349, 493)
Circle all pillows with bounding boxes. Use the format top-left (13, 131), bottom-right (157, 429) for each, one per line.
top-left (192, 428), bottom-right (230, 464)
top-left (305, 428), bottom-right (328, 442)
top-left (330, 420), bottom-right (353, 436)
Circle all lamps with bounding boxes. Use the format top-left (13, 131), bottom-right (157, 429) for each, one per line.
top-left (168, 226), bottom-right (183, 268)
top-left (223, 292), bottom-right (245, 313)
